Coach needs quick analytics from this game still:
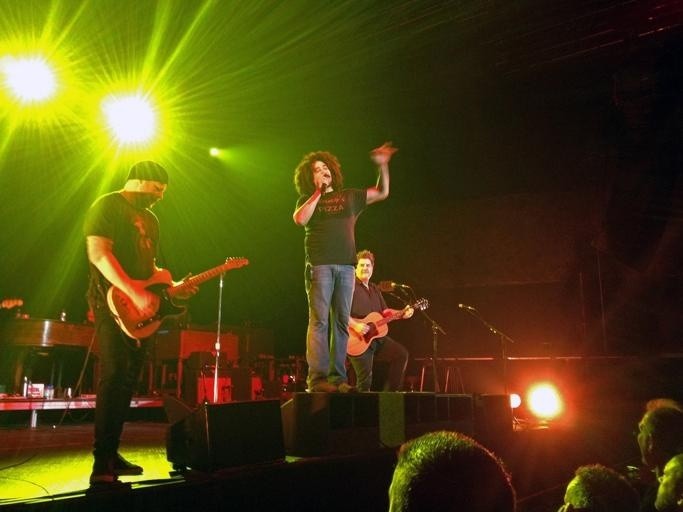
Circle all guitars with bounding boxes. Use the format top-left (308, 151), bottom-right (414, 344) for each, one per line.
top-left (344, 299), bottom-right (430, 358)
top-left (106, 256), bottom-right (251, 340)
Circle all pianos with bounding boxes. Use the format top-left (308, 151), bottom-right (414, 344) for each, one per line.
top-left (7, 318), bottom-right (105, 395)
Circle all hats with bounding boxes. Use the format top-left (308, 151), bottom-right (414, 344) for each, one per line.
top-left (128, 160), bottom-right (169, 184)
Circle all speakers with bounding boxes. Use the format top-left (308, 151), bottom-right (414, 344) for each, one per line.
top-left (436, 393), bottom-right (474, 436)
top-left (281, 389), bottom-right (381, 455)
top-left (167, 400), bottom-right (285, 474)
top-left (380, 391), bottom-right (437, 451)
top-left (473, 392), bottom-right (513, 433)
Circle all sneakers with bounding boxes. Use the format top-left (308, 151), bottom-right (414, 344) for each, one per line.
top-left (90, 454), bottom-right (143, 491)
top-left (307, 380), bottom-right (358, 393)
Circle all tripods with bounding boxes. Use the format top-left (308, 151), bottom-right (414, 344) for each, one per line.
top-left (469, 312), bottom-right (528, 431)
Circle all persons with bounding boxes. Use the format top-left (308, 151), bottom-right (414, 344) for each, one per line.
top-left (625, 398), bottom-right (683, 512)
top-left (292, 141), bottom-right (397, 392)
top-left (85, 160), bottom-right (200, 488)
top-left (554, 463), bottom-right (645, 511)
top-left (345, 249), bottom-right (414, 391)
top-left (651, 452), bottom-right (683, 512)
top-left (387, 431), bottom-right (518, 512)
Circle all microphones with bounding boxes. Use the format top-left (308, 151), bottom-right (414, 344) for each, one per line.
top-left (459, 304), bottom-right (471, 310)
top-left (321, 174), bottom-right (330, 192)
top-left (391, 281), bottom-right (407, 288)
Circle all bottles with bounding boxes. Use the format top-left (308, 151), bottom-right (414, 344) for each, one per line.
top-left (30, 409), bottom-right (37, 427)
top-left (44, 383), bottom-right (54, 397)
top-left (60, 309), bottom-right (66, 321)
top-left (22, 376), bottom-right (32, 398)
top-left (64, 384), bottom-right (72, 399)
